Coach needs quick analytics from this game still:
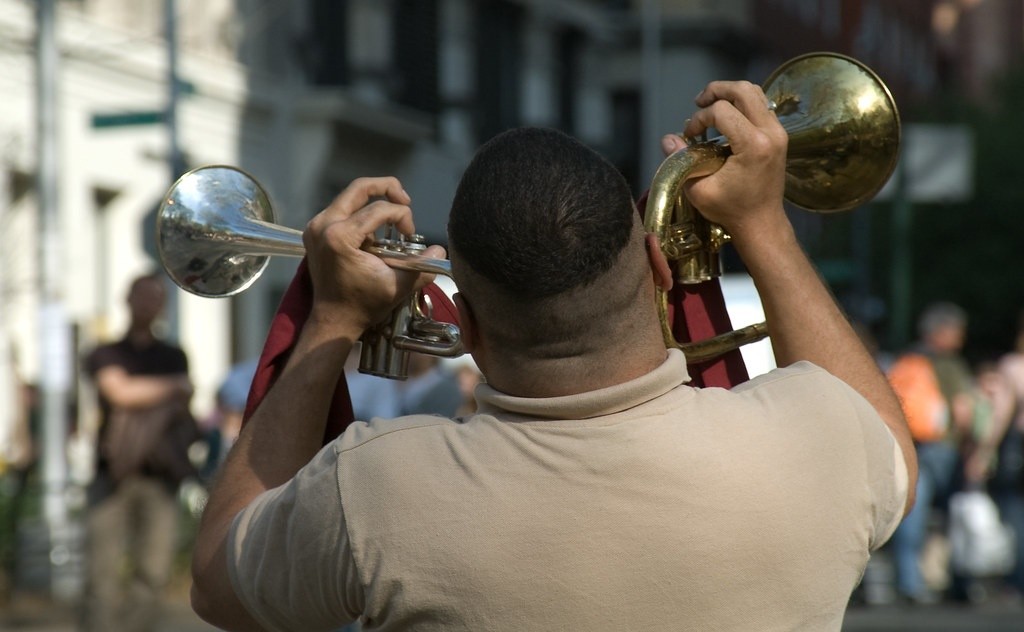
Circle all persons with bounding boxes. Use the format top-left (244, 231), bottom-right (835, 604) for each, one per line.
top-left (189, 81), bottom-right (918, 632)
top-left (0, 270), bottom-right (1024, 631)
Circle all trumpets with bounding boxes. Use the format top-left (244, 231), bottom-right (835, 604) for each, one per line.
top-left (155, 164), bottom-right (470, 381)
top-left (644, 51), bottom-right (904, 367)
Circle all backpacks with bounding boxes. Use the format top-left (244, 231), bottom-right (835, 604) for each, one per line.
top-left (883, 353), bottom-right (950, 443)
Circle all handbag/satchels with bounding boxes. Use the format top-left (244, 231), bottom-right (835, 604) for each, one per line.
top-left (945, 490), bottom-right (1017, 578)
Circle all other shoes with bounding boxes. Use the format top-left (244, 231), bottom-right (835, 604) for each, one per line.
top-left (915, 593), bottom-right (944, 603)
top-left (863, 583), bottom-right (896, 605)
top-left (967, 589), bottom-right (986, 603)
